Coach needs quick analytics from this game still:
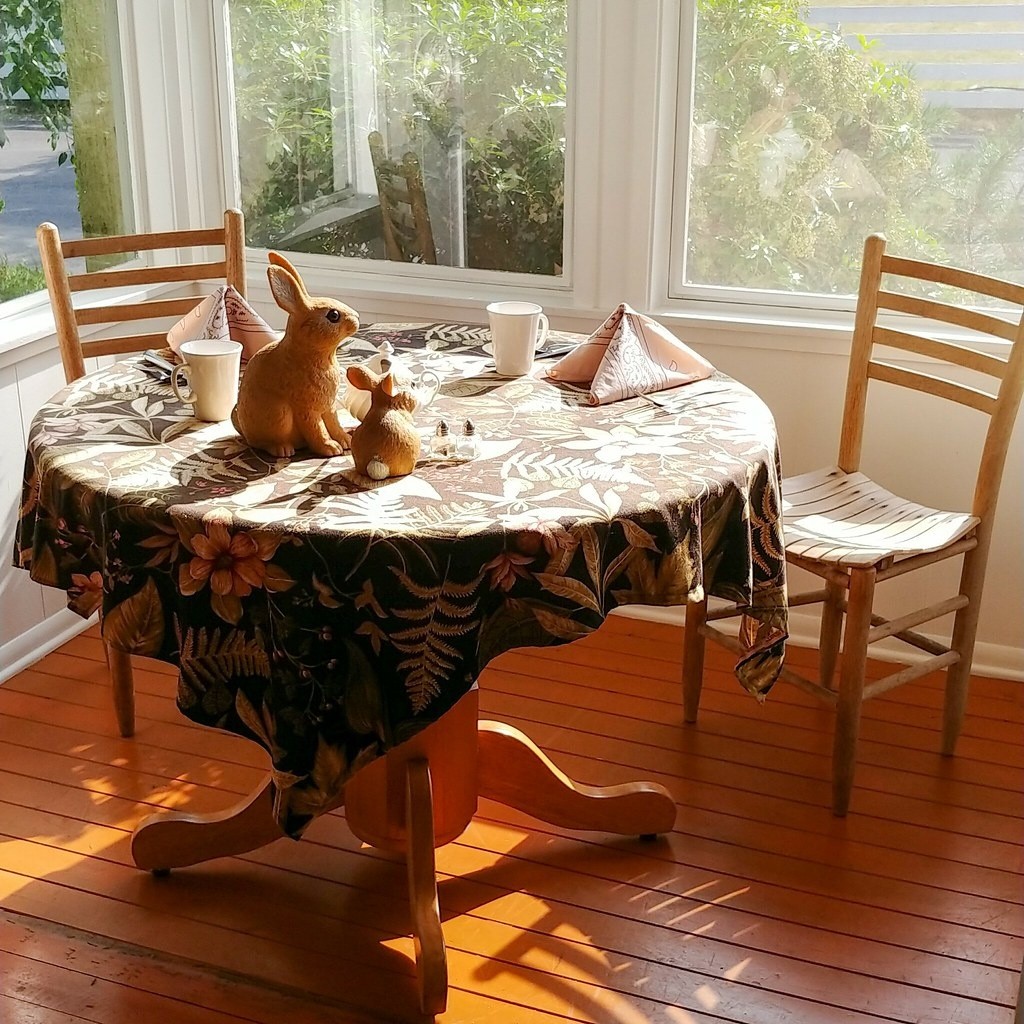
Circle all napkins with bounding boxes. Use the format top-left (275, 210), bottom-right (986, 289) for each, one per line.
top-left (548, 304), bottom-right (714, 406)
top-left (166, 285), bottom-right (283, 359)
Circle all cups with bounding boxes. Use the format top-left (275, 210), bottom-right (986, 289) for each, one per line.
top-left (171, 339), bottom-right (243, 421)
top-left (486, 302), bottom-right (549, 375)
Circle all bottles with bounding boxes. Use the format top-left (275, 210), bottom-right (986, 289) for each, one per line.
top-left (429, 420), bottom-right (457, 458)
top-left (455, 420), bottom-right (483, 461)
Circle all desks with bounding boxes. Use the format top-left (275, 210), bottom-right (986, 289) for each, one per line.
top-left (12, 323), bottom-right (791, 1017)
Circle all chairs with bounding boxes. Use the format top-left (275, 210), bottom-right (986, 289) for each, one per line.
top-left (683, 235), bottom-right (1024, 820)
top-left (367, 130), bottom-right (438, 265)
top-left (34, 206), bottom-right (247, 739)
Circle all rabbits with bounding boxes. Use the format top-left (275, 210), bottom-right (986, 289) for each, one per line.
top-left (231, 251), bottom-right (360, 457)
top-left (346, 365), bottom-right (421, 480)
